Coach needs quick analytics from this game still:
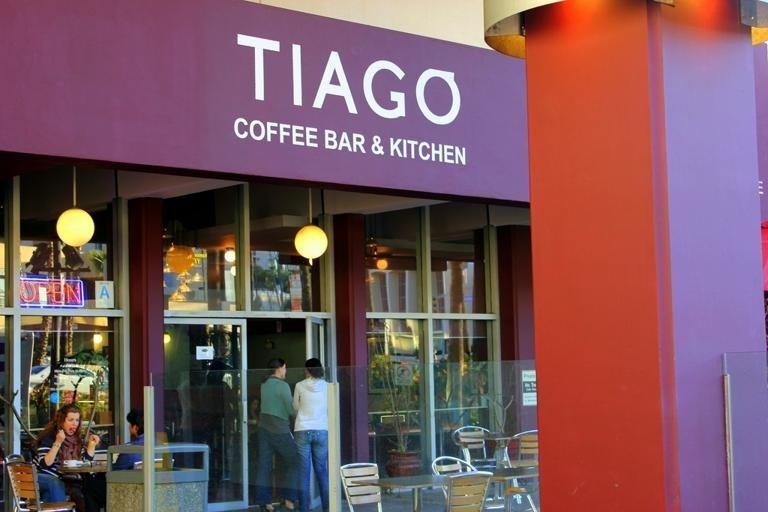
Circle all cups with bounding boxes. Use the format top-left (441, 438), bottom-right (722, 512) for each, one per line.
top-left (64, 460), bottom-right (77, 466)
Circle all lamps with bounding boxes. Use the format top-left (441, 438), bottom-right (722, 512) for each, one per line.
top-left (55, 163), bottom-right (96, 250)
top-left (293, 187), bottom-right (329, 261)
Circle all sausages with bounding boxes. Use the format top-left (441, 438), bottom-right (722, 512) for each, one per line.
top-left (72, 428), bottom-right (76, 432)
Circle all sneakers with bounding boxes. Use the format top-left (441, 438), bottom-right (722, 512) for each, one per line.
top-left (260, 501), bottom-right (295, 512)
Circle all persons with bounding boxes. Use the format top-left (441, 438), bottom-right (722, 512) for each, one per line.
top-left (81, 405), bottom-right (173, 511)
top-left (253, 356), bottom-right (301, 512)
top-left (30, 400), bottom-right (102, 504)
top-left (248, 395), bottom-right (260, 445)
top-left (292, 358), bottom-right (332, 512)
top-left (196, 359), bottom-right (238, 485)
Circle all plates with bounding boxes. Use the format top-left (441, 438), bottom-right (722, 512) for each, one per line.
top-left (64, 465), bottom-right (81, 468)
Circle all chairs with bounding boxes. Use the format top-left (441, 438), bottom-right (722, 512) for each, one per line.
top-left (340, 424), bottom-right (538, 512)
top-left (5, 446), bottom-right (175, 510)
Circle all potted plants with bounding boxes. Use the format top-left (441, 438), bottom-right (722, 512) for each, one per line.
top-left (370, 351), bottom-right (425, 477)
